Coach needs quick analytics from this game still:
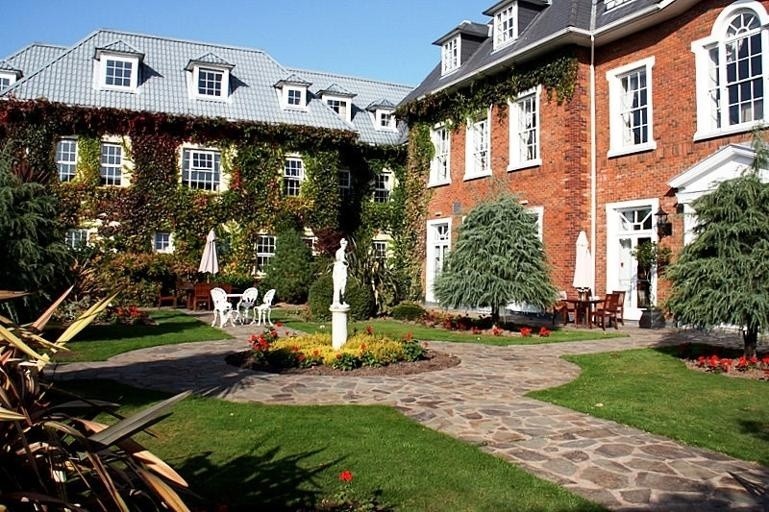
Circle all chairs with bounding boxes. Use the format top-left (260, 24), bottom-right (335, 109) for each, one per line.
top-left (154, 278), bottom-right (277, 330)
top-left (505, 288), bottom-right (626, 330)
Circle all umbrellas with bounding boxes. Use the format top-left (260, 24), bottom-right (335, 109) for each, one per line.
top-left (572, 229), bottom-right (594, 298)
top-left (197, 227), bottom-right (219, 284)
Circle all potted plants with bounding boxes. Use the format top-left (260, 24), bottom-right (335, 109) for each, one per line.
top-left (629, 241), bottom-right (674, 329)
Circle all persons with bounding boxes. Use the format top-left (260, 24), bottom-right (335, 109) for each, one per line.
top-left (331, 237), bottom-right (352, 309)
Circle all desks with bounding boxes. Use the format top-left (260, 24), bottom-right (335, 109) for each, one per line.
top-left (0, 289), bottom-right (37, 324)
